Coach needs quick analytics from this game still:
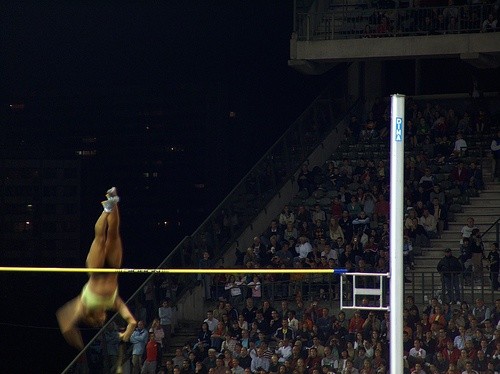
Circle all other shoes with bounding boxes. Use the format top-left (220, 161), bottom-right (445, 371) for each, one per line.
top-left (105, 187), bottom-right (117, 200)
top-left (101, 196), bottom-right (119, 212)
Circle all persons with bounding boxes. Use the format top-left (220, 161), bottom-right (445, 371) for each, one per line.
top-left (88, 210), bottom-right (243, 374)
top-left (55, 187), bottom-right (137, 349)
top-left (160, 296), bottom-right (500, 374)
top-left (197, 251), bottom-right (215, 300)
top-left (213, 85), bottom-right (500, 307)
top-left (485, 242), bottom-right (500, 292)
top-left (129, 299), bottom-right (176, 374)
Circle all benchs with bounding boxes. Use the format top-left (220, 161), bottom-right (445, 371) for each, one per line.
top-left (290, 126), bottom-right (500, 303)
